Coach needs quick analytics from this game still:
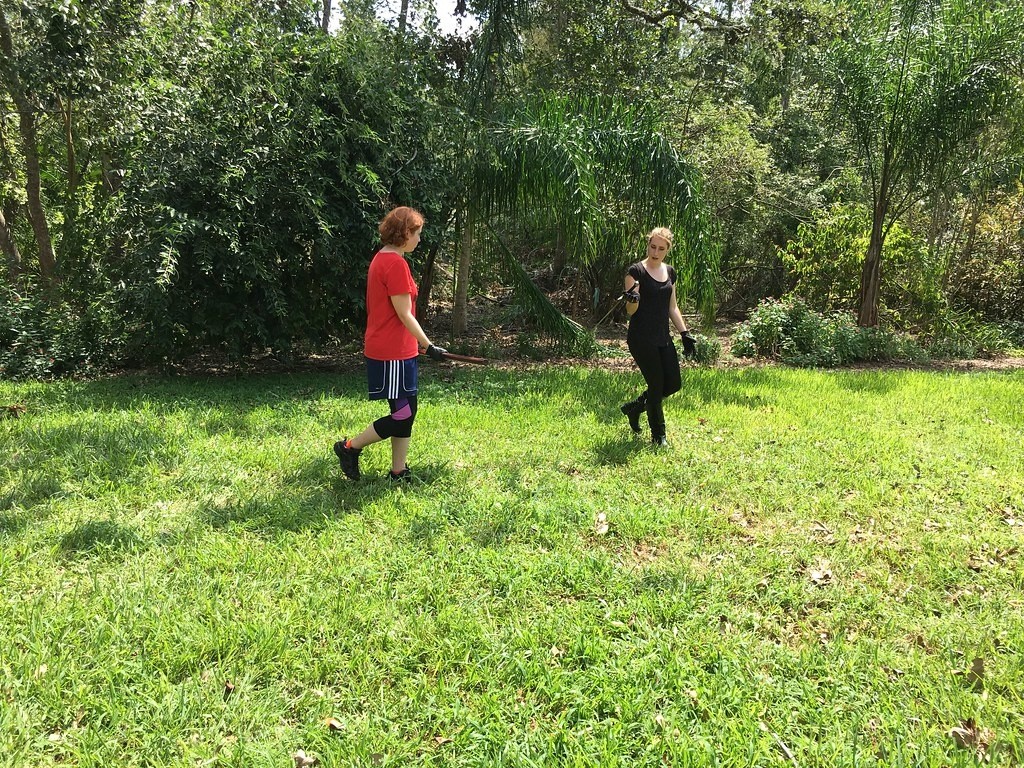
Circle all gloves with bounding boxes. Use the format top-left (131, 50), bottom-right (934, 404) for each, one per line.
top-left (426, 344), bottom-right (449, 362)
top-left (680, 331), bottom-right (697, 357)
top-left (617, 291), bottom-right (640, 302)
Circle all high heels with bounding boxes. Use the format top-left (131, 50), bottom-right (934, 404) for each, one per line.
top-left (652, 434), bottom-right (670, 450)
top-left (621, 398), bottom-right (644, 433)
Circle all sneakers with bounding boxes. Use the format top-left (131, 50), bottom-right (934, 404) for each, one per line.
top-left (334, 440), bottom-right (362, 481)
top-left (389, 462), bottom-right (412, 484)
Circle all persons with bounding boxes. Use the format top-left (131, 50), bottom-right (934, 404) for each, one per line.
top-left (621, 227), bottom-right (698, 451)
top-left (334, 206), bottom-right (447, 486)
